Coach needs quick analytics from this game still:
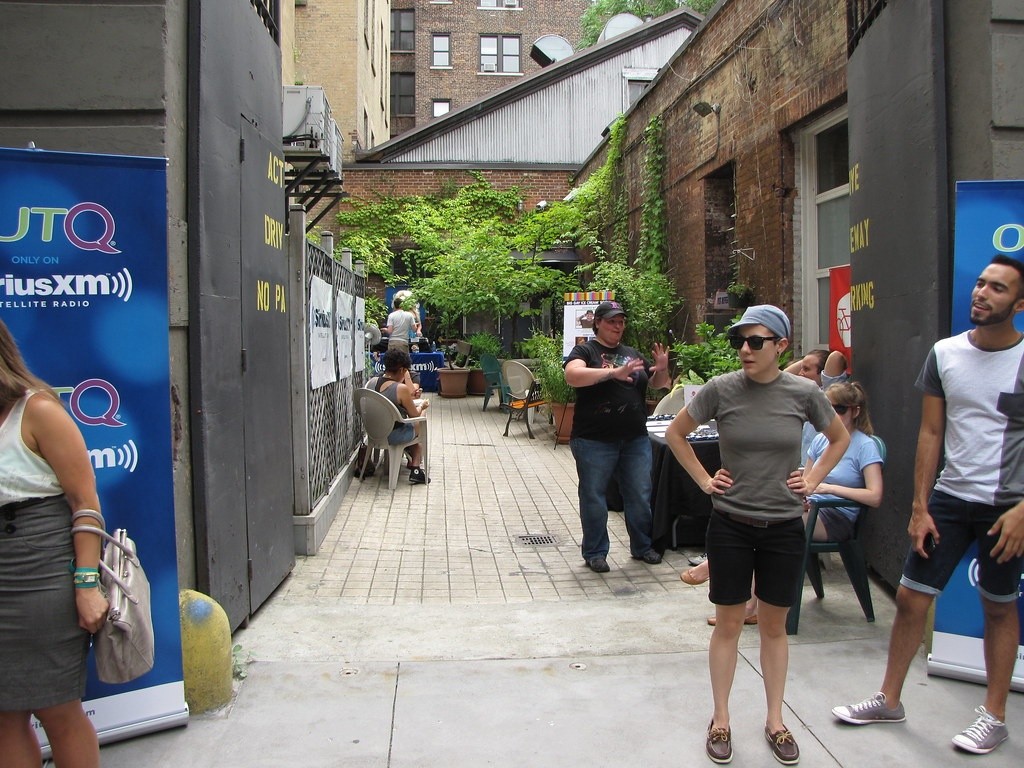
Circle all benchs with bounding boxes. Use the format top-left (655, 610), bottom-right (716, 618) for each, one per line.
top-left (500, 379), bottom-right (553, 440)
top-left (443, 339), bottom-right (473, 368)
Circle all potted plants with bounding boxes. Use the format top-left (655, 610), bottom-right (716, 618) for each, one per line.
top-left (415, 207), bottom-right (567, 396)
top-left (574, 261), bottom-right (684, 415)
top-left (521, 324), bottom-right (577, 445)
top-left (467, 329), bottom-right (504, 396)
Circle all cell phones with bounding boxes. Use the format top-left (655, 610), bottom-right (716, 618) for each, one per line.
top-left (923, 533), bottom-right (936, 554)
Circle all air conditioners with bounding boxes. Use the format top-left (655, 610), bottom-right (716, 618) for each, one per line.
top-left (283, 85), bottom-right (344, 193)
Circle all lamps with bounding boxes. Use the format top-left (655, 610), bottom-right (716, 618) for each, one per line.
top-left (693, 101), bottom-right (723, 118)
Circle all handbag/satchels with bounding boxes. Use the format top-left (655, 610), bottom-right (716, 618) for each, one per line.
top-left (71, 509), bottom-right (155, 684)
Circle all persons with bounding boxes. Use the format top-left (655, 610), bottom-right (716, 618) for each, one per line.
top-left (388, 293), bottom-right (421, 352)
top-left (681, 349), bottom-right (883, 626)
top-left (576, 310), bottom-right (595, 328)
top-left (832, 254), bottom-right (1024, 754)
top-left (563, 302), bottom-right (672, 573)
top-left (0, 318), bottom-right (102, 768)
top-left (665, 304), bottom-right (852, 765)
top-left (365, 349), bottom-right (430, 483)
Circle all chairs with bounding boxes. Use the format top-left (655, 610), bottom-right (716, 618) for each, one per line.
top-left (479, 353), bottom-right (537, 424)
top-left (354, 388), bottom-right (429, 489)
top-left (783, 434), bottom-right (887, 636)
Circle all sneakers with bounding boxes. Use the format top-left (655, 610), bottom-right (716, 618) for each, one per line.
top-left (765, 722), bottom-right (800, 765)
top-left (830, 691), bottom-right (907, 725)
top-left (706, 718), bottom-right (732, 765)
top-left (409, 467), bottom-right (431, 484)
top-left (953, 705), bottom-right (1010, 754)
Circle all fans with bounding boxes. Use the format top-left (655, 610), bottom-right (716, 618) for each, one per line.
top-left (364, 323), bottom-right (381, 380)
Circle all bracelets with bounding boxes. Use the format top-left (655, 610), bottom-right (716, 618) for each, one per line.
top-left (420, 404), bottom-right (424, 410)
top-left (72, 567), bottom-right (97, 587)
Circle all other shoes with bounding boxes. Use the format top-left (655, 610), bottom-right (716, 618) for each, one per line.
top-left (680, 565), bottom-right (710, 586)
top-left (707, 613), bottom-right (758, 626)
top-left (687, 554), bottom-right (708, 566)
top-left (640, 549), bottom-right (662, 564)
top-left (585, 559), bottom-right (610, 573)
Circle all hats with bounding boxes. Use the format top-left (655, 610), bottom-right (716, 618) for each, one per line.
top-left (728, 304), bottom-right (792, 340)
top-left (594, 302), bottom-right (628, 319)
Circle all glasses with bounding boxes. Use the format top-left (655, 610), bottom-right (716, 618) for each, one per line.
top-left (831, 404), bottom-right (856, 416)
top-left (728, 334), bottom-right (780, 351)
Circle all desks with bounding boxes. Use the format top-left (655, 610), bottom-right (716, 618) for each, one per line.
top-left (366, 351), bottom-right (444, 393)
top-left (608, 418), bottom-right (721, 548)
